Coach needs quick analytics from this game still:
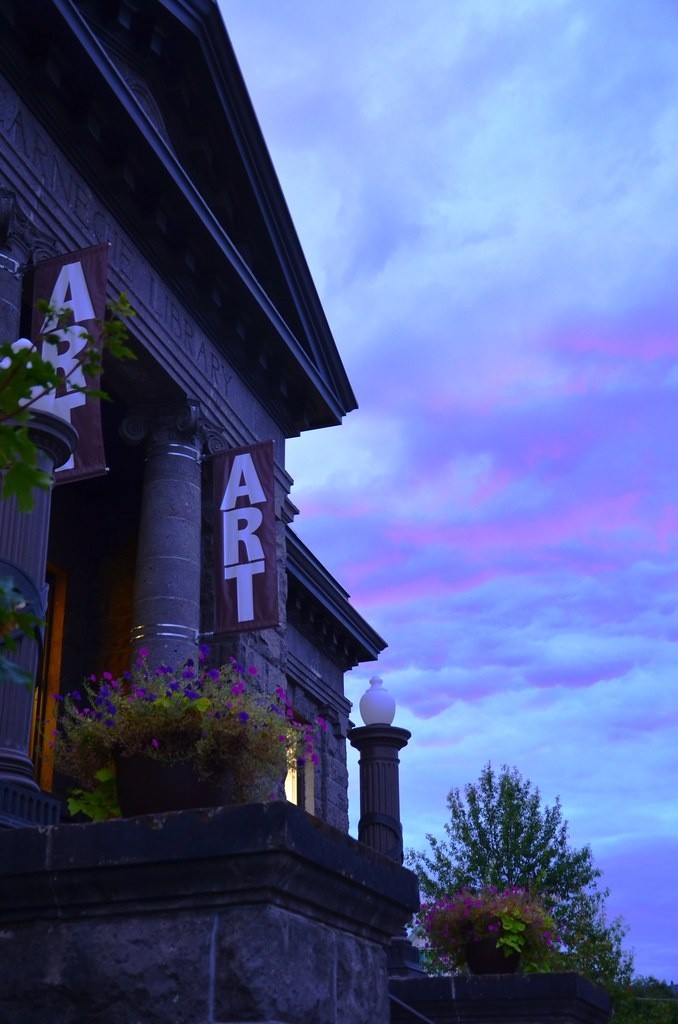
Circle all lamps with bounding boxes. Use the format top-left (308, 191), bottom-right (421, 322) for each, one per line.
top-left (358, 675), bottom-right (396, 726)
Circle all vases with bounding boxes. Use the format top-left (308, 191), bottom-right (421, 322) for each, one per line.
top-left (461, 933), bottom-right (526, 977)
top-left (113, 733), bottom-right (200, 816)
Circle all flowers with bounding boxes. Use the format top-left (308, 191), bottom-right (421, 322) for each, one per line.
top-left (32, 641), bottom-right (326, 803)
top-left (405, 879), bottom-right (571, 979)
